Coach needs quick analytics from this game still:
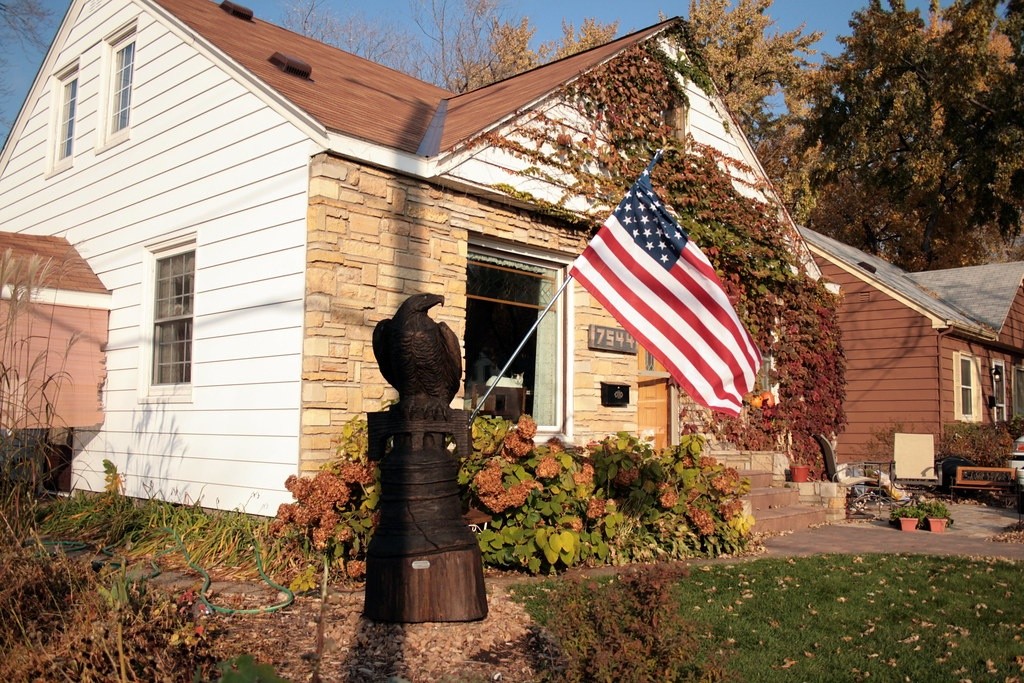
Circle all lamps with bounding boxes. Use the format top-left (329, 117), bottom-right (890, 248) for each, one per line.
top-left (991, 364), bottom-right (1001, 379)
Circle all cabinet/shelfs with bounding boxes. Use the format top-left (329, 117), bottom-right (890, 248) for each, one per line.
top-left (839, 459), bottom-right (894, 520)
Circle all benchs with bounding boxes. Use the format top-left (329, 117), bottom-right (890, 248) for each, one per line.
top-left (949, 465), bottom-right (1017, 508)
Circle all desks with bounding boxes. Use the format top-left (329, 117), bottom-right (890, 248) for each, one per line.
top-left (895, 480), bottom-right (934, 506)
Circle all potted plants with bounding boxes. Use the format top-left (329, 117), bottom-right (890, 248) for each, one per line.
top-left (921, 501), bottom-right (955, 532)
top-left (789, 463), bottom-right (809, 482)
top-left (892, 506), bottom-right (922, 531)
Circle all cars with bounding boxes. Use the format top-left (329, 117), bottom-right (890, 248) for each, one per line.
top-left (1006, 436), bottom-right (1024, 488)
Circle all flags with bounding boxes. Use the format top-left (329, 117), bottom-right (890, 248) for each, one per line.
top-left (568, 158), bottom-right (766, 420)
